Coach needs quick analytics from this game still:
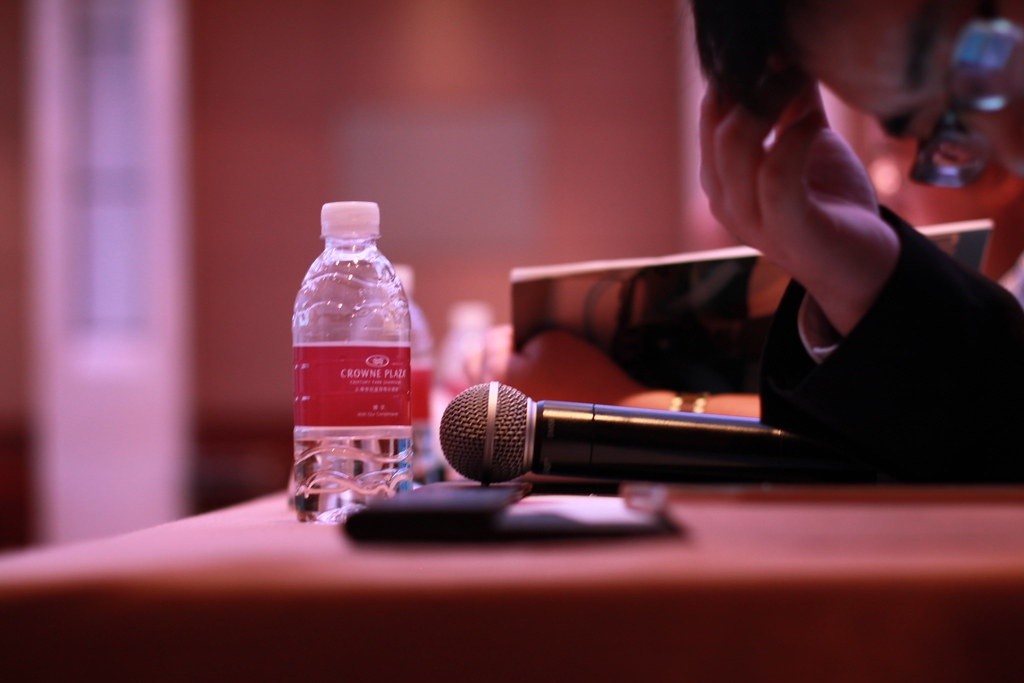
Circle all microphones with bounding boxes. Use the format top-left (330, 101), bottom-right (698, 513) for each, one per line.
top-left (440, 380), bottom-right (807, 484)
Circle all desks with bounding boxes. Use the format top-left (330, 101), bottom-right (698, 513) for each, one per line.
top-left (0, 488), bottom-right (1024, 683)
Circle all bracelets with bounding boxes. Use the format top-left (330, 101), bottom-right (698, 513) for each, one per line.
top-left (669, 384), bottom-right (708, 416)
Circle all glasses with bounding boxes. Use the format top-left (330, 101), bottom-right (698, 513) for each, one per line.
top-left (911, 0), bottom-right (999, 189)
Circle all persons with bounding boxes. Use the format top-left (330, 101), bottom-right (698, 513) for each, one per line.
top-left (501, 0), bottom-right (1024, 488)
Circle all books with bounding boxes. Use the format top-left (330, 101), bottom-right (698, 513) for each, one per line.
top-left (507, 220), bottom-right (993, 354)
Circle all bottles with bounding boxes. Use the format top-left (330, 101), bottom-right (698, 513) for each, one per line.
top-left (290, 201), bottom-right (412, 524)
top-left (484, 324), bottom-right (512, 385)
top-left (353, 264), bottom-right (437, 489)
top-left (425, 301), bottom-right (489, 465)
